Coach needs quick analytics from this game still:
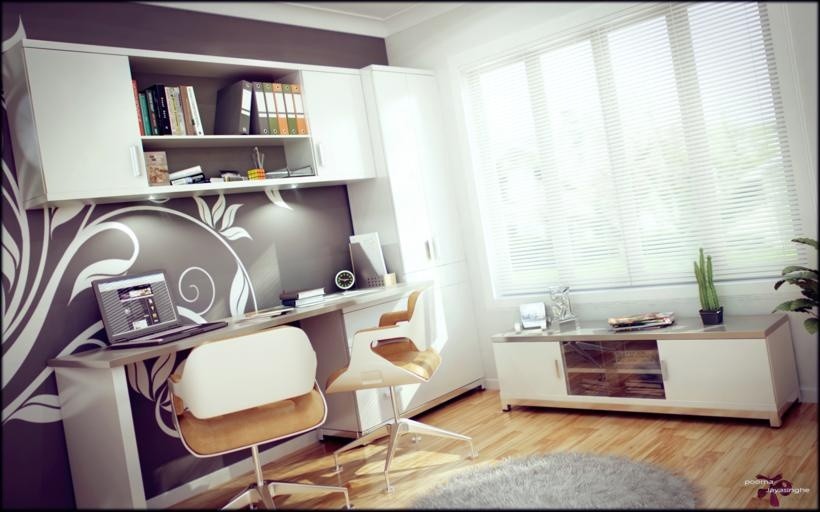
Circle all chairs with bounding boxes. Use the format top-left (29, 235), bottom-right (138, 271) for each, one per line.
top-left (166, 325), bottom-right (353, 511)
top-left (325, 289), bottom-right (479, 495)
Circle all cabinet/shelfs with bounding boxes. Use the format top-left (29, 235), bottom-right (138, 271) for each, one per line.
top-left (2, 39), bottom-right (378, 212)
top-left (490, 312), bottom-right (803, 428)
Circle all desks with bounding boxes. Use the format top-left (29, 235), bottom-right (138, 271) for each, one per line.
top-left (45, 279), bottom-right (433, 511)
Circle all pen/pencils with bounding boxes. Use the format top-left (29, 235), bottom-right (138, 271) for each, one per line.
top-left (249, 146), bottom-right (266, 169)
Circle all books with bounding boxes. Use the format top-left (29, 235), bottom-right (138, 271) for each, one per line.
top-left (279, 287), bottom-right (326, 308)
top-left (132, 80), bottom-right (204, 136)
top-left (579, 374), bottom-right (664, 395)
top-left (169, 165), bottom-right (225, 185)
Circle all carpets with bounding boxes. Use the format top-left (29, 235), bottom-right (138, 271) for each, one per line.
top-left (408, 451), bottom-right (705, 511)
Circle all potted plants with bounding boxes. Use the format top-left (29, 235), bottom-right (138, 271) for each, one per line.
top-left (693, 248), bottom-right (724, 325)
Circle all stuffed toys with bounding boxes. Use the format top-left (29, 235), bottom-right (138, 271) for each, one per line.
top-left (247, 168), bottom-right (265, 180)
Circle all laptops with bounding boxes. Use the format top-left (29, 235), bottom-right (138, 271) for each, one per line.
top-left (92, 269), bottom-right (228, 350)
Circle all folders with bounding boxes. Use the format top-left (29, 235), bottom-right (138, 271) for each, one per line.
top-left (215, 81), bottom-right (309, 133)
